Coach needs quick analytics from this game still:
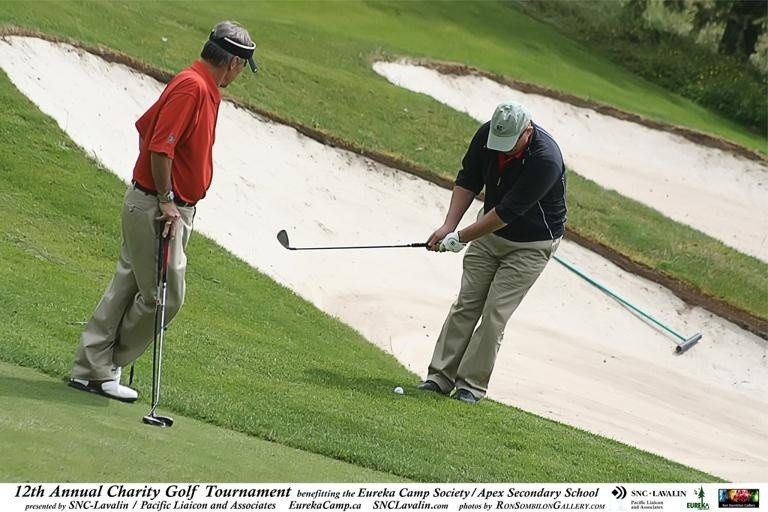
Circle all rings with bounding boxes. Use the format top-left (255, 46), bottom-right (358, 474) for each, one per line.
top-left (164, 221), bottom-right (171, 225)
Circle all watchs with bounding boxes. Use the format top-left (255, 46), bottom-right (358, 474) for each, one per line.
top-left (157, 191), bottom-right (176, 203)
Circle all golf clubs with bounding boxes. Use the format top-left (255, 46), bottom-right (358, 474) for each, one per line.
top-left (275, 230), bottom-right (443, 250)
top-left (141, 216), bottom-right (175, 427)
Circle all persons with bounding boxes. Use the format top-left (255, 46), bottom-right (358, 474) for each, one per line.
top-left (415, 100), bottom-right (567, 408)
top-left (67, 19), bottom-right (257, 400)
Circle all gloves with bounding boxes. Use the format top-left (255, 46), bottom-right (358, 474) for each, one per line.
top-left (437, 229), bottom-right (468, 253)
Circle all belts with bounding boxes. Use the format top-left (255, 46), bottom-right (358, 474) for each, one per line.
top-left (132, 179), bottom-right (196, 209)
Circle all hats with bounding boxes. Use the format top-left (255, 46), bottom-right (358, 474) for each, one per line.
top-left (209, 29), bottom-right (259, 74)
top-left (486, 101), bottom-right (532, 153)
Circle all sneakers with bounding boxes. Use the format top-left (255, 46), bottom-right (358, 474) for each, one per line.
top-left (67, 376), bottom-right (139, 403)
top-left (416, 380), bottom-right (441, 394)
top-left (451, 389), bottom-right (480, 405)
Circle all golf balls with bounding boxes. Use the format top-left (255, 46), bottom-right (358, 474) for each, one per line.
top-left (394, 387), bottom-right (404, 393)
